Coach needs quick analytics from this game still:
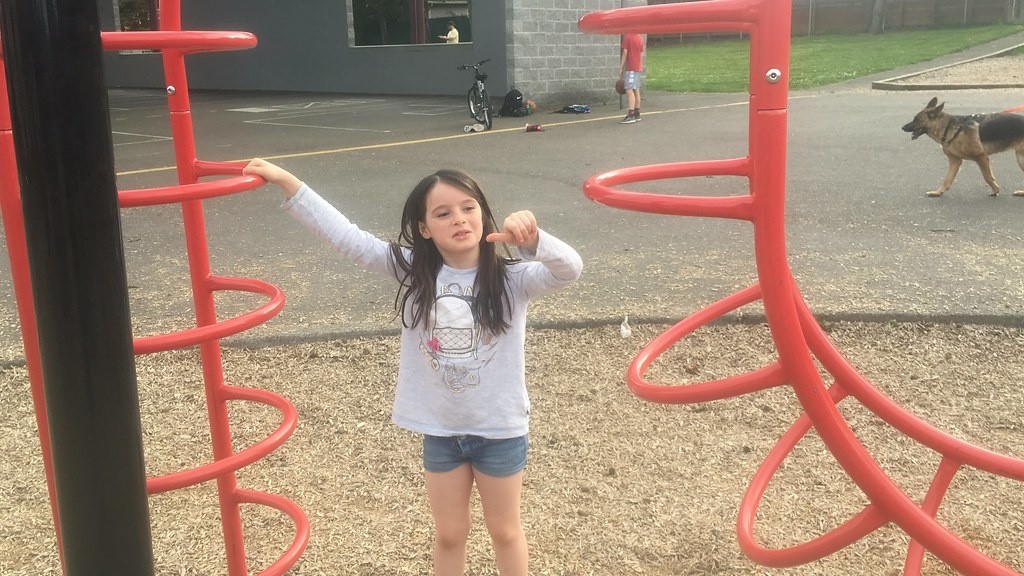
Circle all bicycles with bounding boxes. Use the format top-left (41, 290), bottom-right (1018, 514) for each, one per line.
top-left (457, 58), bottom-right (497, 130)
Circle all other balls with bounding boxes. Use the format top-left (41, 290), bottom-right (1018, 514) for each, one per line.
top-left (524, 100), bottom-right (536, 112)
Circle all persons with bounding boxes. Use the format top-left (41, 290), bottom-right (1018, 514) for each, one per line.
top-left (243, 158), bottom-right (583, 576)
top-left (437, 21), bottom-right (459, 44)
top-left (619, 34), bottom-right (645, 124)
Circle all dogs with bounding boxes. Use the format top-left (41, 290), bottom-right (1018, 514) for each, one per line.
top-left (901, 96), bottom-right (1023, 197)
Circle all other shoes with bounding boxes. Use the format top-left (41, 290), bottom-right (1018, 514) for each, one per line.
top-left (464, 124), bottom-right (484, 133)
top-left (526, 123), bottom-right (542, 132)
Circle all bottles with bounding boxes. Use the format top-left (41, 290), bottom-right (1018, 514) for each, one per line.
top-left (525, 104), bottom-right (529, 108)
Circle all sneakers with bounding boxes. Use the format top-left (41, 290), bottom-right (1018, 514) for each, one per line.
top-left (619, 115), bottom-right (636, 123)
top-left (635, 113), bottom-right (642, 121)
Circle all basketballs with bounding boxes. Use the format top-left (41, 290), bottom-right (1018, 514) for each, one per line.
top-left (615, 80), bottom-right (626, 94)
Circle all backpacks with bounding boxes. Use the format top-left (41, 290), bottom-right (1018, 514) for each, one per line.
top-left (497, 86), bottom-right (523, 116)
